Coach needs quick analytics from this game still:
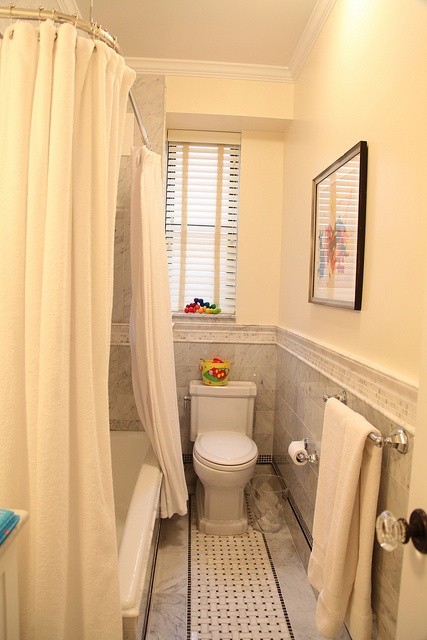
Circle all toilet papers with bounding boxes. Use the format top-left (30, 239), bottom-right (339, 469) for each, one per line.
top-left (288, 441), bottom-right (308, 466)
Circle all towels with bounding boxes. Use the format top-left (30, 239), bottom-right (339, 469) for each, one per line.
top-left (308, 397), bottom-right (382, 640)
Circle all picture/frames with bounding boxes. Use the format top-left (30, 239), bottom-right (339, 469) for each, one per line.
top-left (307, 139), bottom-right (369, 311)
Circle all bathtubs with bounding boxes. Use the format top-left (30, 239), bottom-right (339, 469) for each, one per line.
top-left (108, 432), bottom-right (169, 640)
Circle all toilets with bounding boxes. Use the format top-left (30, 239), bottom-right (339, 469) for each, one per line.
top-left (189, 380), bottom-right (258, 536)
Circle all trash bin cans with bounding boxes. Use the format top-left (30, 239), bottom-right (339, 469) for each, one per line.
top-left (248, 475), bottom-right (290, 533)
